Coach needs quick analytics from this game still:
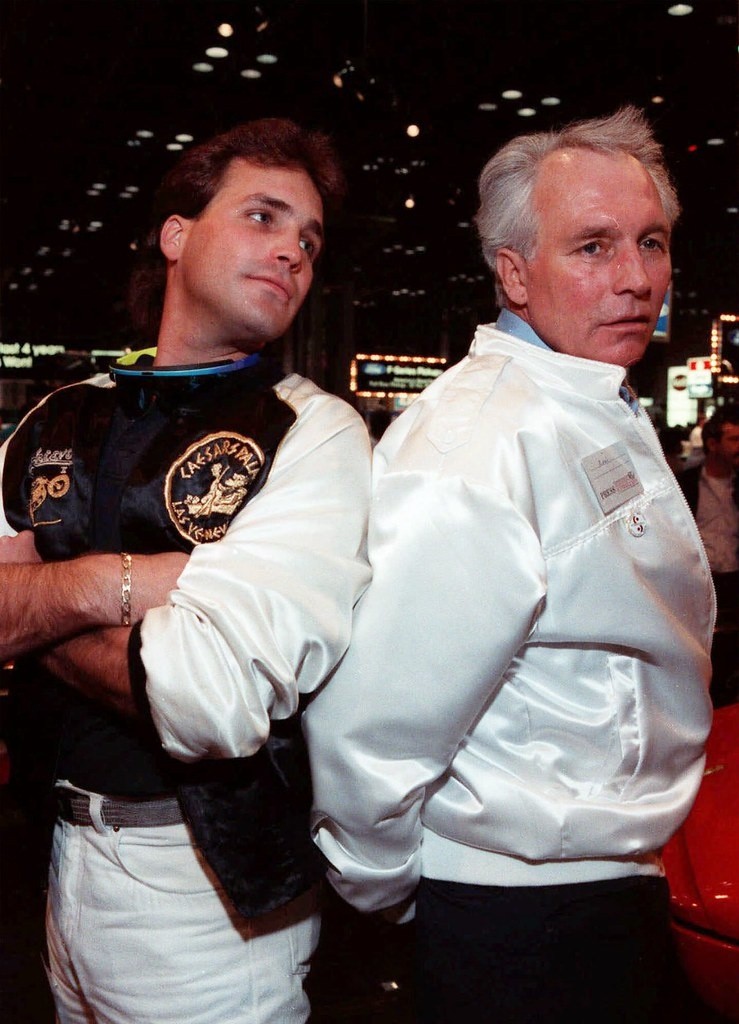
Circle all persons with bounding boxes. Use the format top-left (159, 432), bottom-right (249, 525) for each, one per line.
top-left (1, 118), bottom-right (374, 1024)
top-left (303, 104), bottom-right (715, 1024)
top-left (675, 402), bottom-right (739, 706)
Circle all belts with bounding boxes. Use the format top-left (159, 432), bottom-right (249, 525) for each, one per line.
top-left (53, 787), bottom-right (182, 829)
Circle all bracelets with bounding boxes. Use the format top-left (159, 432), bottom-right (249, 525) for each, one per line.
top-left (120, 552), bottom-right (134, 626)
